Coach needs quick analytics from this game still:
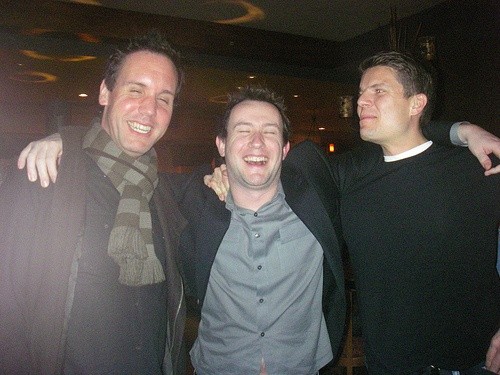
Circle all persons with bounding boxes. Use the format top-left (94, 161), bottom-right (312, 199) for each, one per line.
top-left (203, 50), bottom-right (499, 375)
top-left (0, 32), bottom-right (187, 375)
top-left (17, 85), bottom-right (500, 375)
